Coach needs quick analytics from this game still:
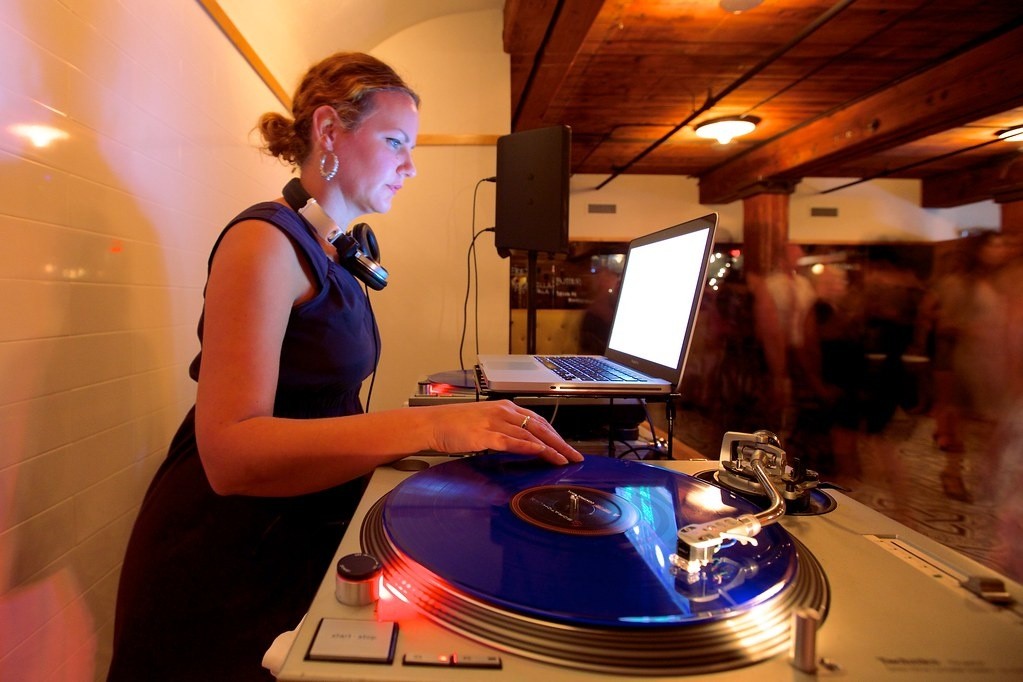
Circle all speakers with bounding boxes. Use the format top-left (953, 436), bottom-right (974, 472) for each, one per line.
top-left (494, 125), bottom-right (573, 253)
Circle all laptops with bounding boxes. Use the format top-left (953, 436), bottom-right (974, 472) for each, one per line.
top-left (473, 211), bottom-right (719, 394)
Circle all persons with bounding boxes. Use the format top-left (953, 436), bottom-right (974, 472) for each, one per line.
top-left (106, 53), bottom-right (584, 682)
top-left (711, 226), bottom-right (1023, 582)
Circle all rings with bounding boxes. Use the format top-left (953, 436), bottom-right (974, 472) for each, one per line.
top-left (521, 416), bottom-right (530, 428)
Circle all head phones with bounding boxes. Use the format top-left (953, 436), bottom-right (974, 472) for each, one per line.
top-left (282, 177), bottom-right (389, 292)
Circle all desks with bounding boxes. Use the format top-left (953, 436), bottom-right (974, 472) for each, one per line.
top-left (279, 421), bottom-right (1022, 681)
